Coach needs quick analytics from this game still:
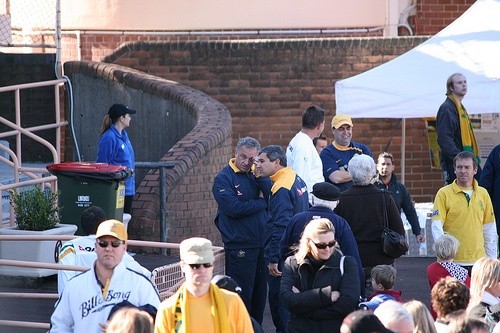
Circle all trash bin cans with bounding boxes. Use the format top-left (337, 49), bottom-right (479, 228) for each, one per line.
top-left (45, 161), bottom-right (133, 237)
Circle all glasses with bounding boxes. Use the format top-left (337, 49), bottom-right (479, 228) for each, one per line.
top-left (187, 263), bottom-right (213, 270)
top-left (96, 239), bottom-right (124, 248)
top-left (307, 238), bottom-right (336, 249)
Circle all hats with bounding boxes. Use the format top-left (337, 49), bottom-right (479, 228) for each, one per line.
top-left (359, 294), bottom-right (396, 311)
top-left (212, 275), bottom-right (242, 293)
top-left (95, 219), bottom-right (127, 243)
top-left (310, 182), bottom-right (341, 201)
top-left (340, 309), bottom-right (395, 333)
top-left (108, 104), bottom-right (136, 119)
top-left (180, 237), bottom-right (215, 264)
top-left (373, 300), bottom-right (414, 333)
top-left (331, 114), bottom-right (353, 129)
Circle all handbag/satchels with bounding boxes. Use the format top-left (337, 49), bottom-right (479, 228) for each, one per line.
top-left (381, 189), bottom-right (409, 258)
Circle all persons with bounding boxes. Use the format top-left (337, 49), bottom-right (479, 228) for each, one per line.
top-left (280, 182), bottom-right (363, 292)
top-left (426, 234), bottom-right (470, 290)
top-left (212, 137), bottom-right (267, 333)
top-left (49, 219), bottom-right (162, 333)
top-left (371, 152), bottom-right (425, 243)
top-left (212, 275), bottom-right (264, 333)
top-left (479, 144), bottom-right (500, 235)
top-left (286, 106), bottom-right (327, 205)
top-left (332, 154), bottom-right (405, 298)
top-left (280, 218), bottom-right (360, 333)
top-left (340, 257), bottom-right (500, 333)
top-left (256, 145), bottom-right (308, 333)
top-left (319, 113), bottom-right (372, 193)
top-left (106, 308), bottom-right (154, 333)
top-left (435, 73), bottom-right (482, 185)
top-left (154, 237), bottom-right (254, 333)
top-left (431, 151), bottom-right (499, 277)
top-left (58, 207), bottom-right (151, 299)
top-left (95, 104), bottom-right (136, 225)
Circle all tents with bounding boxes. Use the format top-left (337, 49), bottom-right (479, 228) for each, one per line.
top-left (335, 0), bottom-right (500, 184)
